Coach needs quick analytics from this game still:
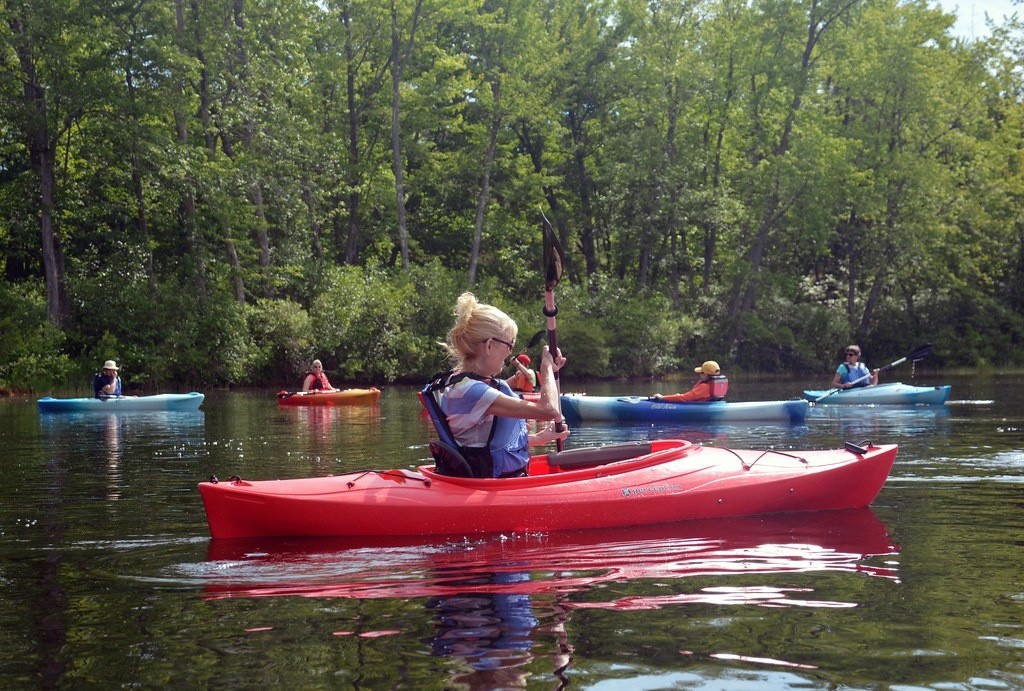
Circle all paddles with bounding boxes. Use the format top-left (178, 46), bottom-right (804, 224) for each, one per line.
top-left (98, 391), bottom-right (138, 401)
top-left (491, 330), bottom-right (546, 378)
top-left (787, 341), bottom-right (933, 404)
top-left (537, 204), bottom-right (567, 452)
top-left (276, 388), bottom-right (341, 400)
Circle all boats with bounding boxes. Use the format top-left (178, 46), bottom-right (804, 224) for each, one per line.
top-left (561, 399), bottom-right (810, 422)
top-left (36, 390), bottom-right (208, 411)
top-left (197, 438), bottom-right (900, 540)
top-left (802, 381), bottom-right (952, 404)
top-left (414, 391), bottom-right (542, 408)
top-left (273, 385), bottom-right (380, 406)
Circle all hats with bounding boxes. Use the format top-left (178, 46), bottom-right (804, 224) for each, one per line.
top-left (517, 355), bottom-right (530, 366)
top-left (103, 360), bottom-right (119, 370)
top-left (695, 360), bottom-right (720, 376)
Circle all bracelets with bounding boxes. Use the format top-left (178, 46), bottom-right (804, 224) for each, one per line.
top-left (515, 362), bottom-right (521, 368)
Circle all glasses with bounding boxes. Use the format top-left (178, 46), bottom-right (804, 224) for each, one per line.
top-left (482, 337), bottom-right (515, 354)
top-left (314, 366), bottom-right (321, 368)
top-left (845, 353), bottom-right (857, 356)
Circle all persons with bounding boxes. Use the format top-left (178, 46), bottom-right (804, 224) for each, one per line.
top-left (93, 360), bottom-right (119, 399)
top-left (432, 291), bottom-right (571, 480)
top-left (654, 360), bottom-right (721, 404)
top-left (831, 345), bottom-right (880, 389)
top-left (303, 359), bottom-right (340, 394)
top-left (505, 354), bottom-right (537, 393)
